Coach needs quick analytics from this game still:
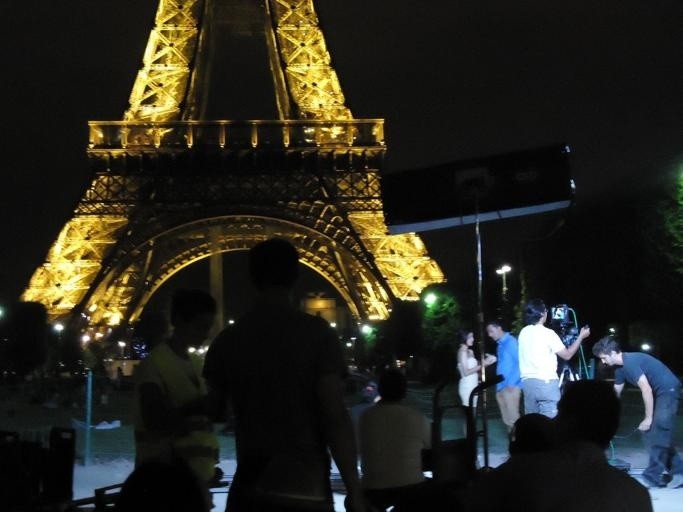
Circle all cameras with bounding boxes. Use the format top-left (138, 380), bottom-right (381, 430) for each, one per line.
top-left (552, 304), bottom-right (568, 323)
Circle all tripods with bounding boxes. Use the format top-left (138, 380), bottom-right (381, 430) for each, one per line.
top-left (559, 367), bottom-right (580, 388)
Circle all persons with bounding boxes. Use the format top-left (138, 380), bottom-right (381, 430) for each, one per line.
top-left (592, 334), bottom-right (682, 489)
top-left (516, 296), bottom-right (592, 419)
top-left (0, 299), bottom-right (89, 511)
top-left (474, 411), bottom-right (558, 485)
top-left (471, 379), bottom-right (653, 511)
top-left (132, 285), bottom-right (232, 511)
top-left (203, 235), bottom-right (379, 511)
top-left (358, 368), bottom-right (438, 512)
top-left (114, 453), bottom-right (212, 512)
top-left (481, 316), bottom-right (522, 441)
top-left (391, 482), bottom-right (466, 512)
top-left (452, 324), bottom-right (482, 441)
